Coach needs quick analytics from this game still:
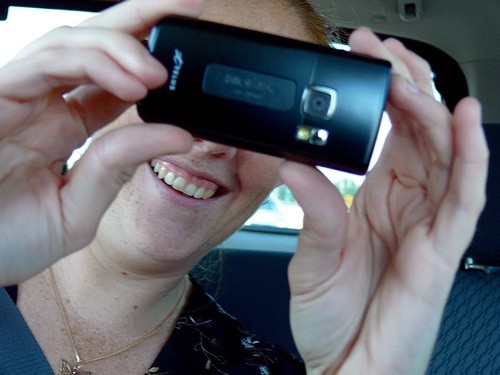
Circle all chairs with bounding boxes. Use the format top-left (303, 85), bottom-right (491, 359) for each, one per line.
top-left (424, 124), bottom-right (500, 375)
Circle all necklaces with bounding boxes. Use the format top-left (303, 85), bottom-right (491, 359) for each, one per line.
top-left (48, 265), bottom-right (186, 375)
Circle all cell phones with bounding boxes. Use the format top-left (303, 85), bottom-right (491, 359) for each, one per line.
top-left (136, 14), bottom-right (392, 176)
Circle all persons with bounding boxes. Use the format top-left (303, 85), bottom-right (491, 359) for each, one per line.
top-left (0, 1), bottom-right (490, 375)
